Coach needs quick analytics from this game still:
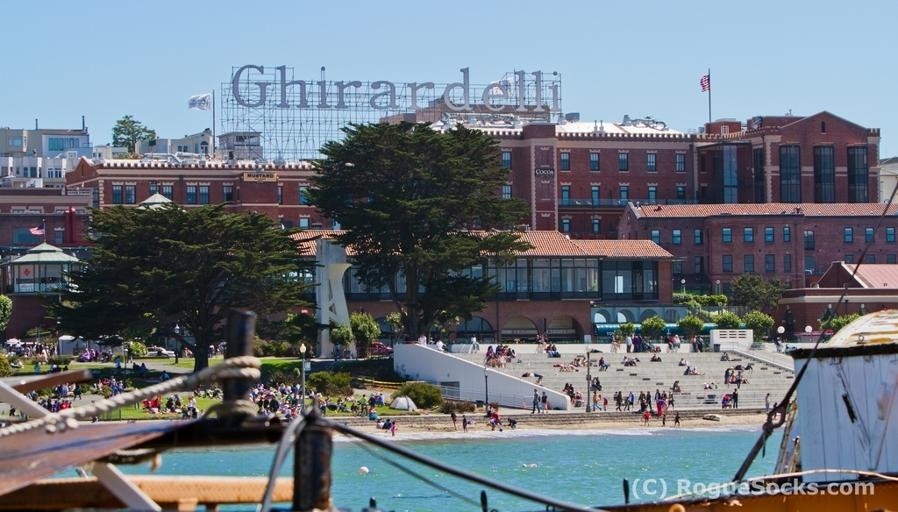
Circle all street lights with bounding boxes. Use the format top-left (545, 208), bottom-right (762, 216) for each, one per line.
top-left (585, 343), bottom-right (591, 412)
top-left (680, 279), bottom-right (686, 293)
top-left (123, 348), bottom-right (129, 388)
top-left (299, 343), bottom-right (306, 413)
top-left (715, 280), bottom-right (720, 295)
top-left (777, 324), bottom-right (813, 352)
top-left (485, 372), bottom-right (488, 410)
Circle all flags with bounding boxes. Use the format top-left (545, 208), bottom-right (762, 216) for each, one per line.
top-left (185, 93), bottom-right (211, 111)
top-left (29, 222), bottom-right (44, 236)
top-left (701, 74), bottom-right (709, 91)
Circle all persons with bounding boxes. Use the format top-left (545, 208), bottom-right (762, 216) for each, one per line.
top-left (0, 327), bottom-right (793, 436)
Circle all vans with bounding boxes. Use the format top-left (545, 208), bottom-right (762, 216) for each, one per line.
top-left (356, 341), bottom-right (393, 357)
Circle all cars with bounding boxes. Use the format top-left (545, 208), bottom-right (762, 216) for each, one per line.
top-left (143, 347), bottom-right (176, 358)
top-left (12, 342), bottom-right (51, 356)
